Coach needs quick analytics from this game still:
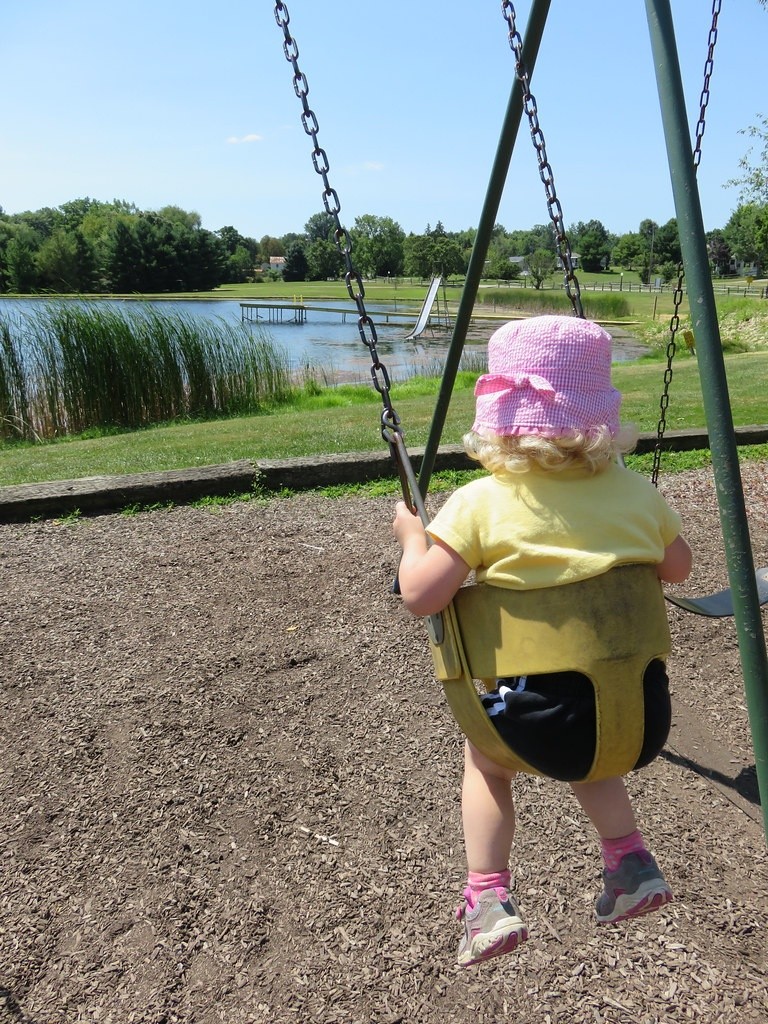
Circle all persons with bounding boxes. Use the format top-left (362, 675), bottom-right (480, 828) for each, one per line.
top-left (392, 314), bottom-right (692, 967)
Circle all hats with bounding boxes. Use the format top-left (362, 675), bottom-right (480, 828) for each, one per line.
top-left (471, 314), bottom-right (621, 438)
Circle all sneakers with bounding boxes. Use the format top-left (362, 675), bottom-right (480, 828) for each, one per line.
top-left (455, 886), bottom-right (528, 967)
top-left (593, 849), bottom-right (674, 923)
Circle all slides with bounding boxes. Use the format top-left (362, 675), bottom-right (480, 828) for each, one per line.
top-left (404, 276), bottom-right (442, 340)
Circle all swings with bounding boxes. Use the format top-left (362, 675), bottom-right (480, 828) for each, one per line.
top-left (272, 0), bottom-right (723, 788)
top-left (500, 0), bottom-right (768, 619)
